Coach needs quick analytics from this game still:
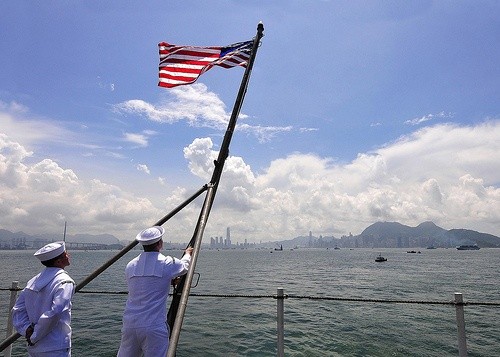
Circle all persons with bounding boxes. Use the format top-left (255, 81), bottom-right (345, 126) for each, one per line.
top-left (116, 225), bottom-right (195, 357)
top-left (11, 240), bottom-right (77, 357)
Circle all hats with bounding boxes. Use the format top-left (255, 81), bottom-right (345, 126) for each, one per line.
top-left (33, 240), bottom-right (66, 262)
top-left (135, 226), bottom-right (166, 246)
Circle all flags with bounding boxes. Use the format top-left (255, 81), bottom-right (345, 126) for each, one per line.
top-left (158, 37), bottom-right (257, 88)
